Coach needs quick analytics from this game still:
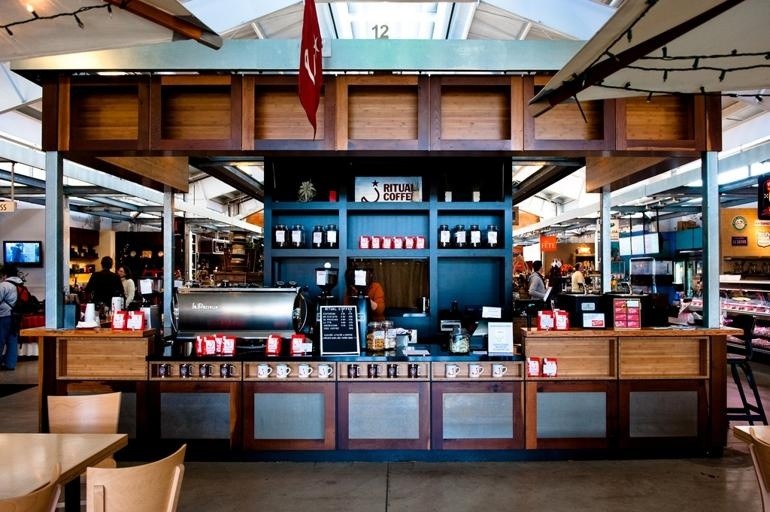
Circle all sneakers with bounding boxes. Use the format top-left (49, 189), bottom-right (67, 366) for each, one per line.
top-left (0, 365), bottom-right (16, 372)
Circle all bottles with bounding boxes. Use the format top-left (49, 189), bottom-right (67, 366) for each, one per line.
top-left (438, 224), bottom-right (500, 247)
top-left (367, 323), bottom-right (384, 353)
top-left (449, 326), bottom-right (470, 356)
top-left (734, 260), bottom-right (770, 273)
top-left (70, 264), bottom-right (93, 273)
top-left (611, 276), bottom-right (617, 292)
top-left (411, 187), bottom-right (420, 203)
top-left (396, 334), bottom-right (408, 356)
top-left (274, 224), bottom-right (339, 248)
top-left (381, 320), bottom-right (396, 350)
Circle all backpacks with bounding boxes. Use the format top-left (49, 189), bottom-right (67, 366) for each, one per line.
top-left (5, 278), bottom-right (36, 316)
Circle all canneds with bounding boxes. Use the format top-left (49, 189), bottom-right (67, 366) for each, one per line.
top-left (271, 223), bottom-right (503, 249)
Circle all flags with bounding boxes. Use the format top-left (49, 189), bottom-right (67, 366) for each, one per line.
top-left (296, 1), bottom-right (325, 143)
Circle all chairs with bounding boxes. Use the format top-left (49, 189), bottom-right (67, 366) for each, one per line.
top-left (747, 427), bottom-right (770, 511)
top-left (0, 462), bottom-right (64, 511)
top-left (85, 443), bottom-right (188, 511)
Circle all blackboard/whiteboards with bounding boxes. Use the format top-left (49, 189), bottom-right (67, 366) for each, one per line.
top-left (320, 306), bottom-right (360, 355)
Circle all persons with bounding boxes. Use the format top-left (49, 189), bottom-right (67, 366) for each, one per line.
top-left (83, 256), bottom-right (127, 312)
top-left (115, 264), bottom-right (136, 310)
top-left (571, 261), bottom-right (585, 294)
top-left (527, 260), bottom-right (547, 302)
top-left (342, 262), bottom-right (386, 323)
top-left (0, 262), bottom-right (25, 371)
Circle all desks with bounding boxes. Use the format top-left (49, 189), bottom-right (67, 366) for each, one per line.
top-left (0, 431), bottom-right (128, 511)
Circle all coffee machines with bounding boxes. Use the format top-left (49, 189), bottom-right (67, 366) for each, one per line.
top-left (629, 257), bottom-right (674, 330)
top-left (347, 267), bottom-right (379, 350)
top-left (312, 268), bottom-right (341, 349)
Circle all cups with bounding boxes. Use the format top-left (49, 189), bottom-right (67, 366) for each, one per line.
top-left (179, 363), bottom-right (195, 378)
top-left (445, 364), bottom-right (461, 378)
top-left (317, 364), bottom-right (333, 378)
top-left (472, 191), bottom-right (481, 204)
top-left (159, 363), bottom-right (174, 378)
top-left (275, 363), bottom-right (292, 378)
top-left (329, 190), bottom-right (337, 202)
top-left (347, 364), bottom-right (360, 378)
top-left (257, 363), bottom-right (273, 378)
top-left (199, 364), bottom-right (215, 378)
top-left (366, 362), bottom-right (382, 378)
top-left (63, 305), bottom-right (79, 328)
top-left (111, 297), bottom-right (123, 315)
top-left (444, 191), bottom-right (452, 202)
top-left (408, 364), bottom-right (421, 378)
top-left (490, 362), bottom-right (507, 379)
top-left (467, 364), bottom-right (484, 378)
top-left (220, 364), bottom-right (237, 378)
top-left (298, 364), bottom-right (313, 378)
top-left (83, 303), bottom-right (96, 322)
top-left (385, 362), bottom-right (398, 378)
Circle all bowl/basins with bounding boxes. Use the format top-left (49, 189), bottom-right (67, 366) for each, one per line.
top-left (677, 221), bottom-right (697, 230)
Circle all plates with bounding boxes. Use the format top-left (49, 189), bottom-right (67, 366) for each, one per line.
top-left (720, 275), bottom-right (741, 282)
top-left (733, 216), bottom-right (747, 231)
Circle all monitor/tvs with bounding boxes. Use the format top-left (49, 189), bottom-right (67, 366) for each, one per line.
top-left (2, 240), bottom-right (43, 268)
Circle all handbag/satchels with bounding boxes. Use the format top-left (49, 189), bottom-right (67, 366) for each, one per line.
top-left (126, 289), bottom-right (142, 311)
